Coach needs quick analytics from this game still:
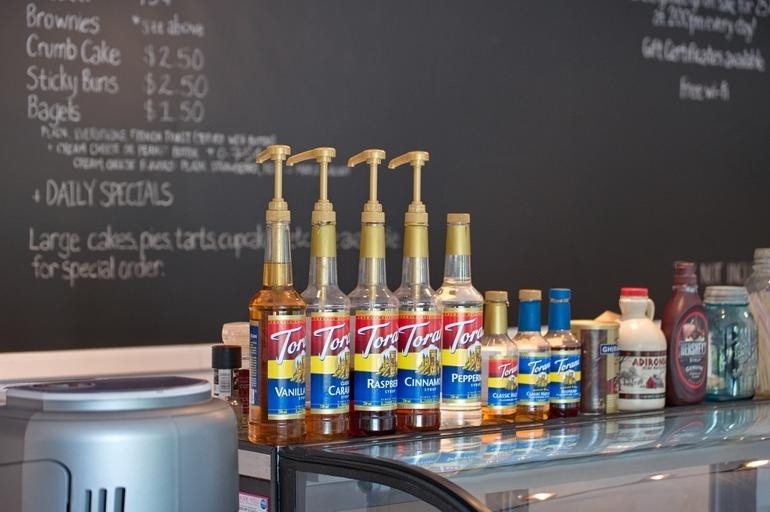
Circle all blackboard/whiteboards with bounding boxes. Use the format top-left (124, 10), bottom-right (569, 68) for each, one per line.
top-left (0, 0), bottom-right (770, 355)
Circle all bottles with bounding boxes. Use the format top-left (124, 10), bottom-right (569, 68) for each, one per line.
top-left (702, 285), bottom-right (759, 403)
top-left (745, 248), bottom-right (770, 400)
top-left (660, 260), bottom-right (709, 405)
top-left (481, 290), bottom-right (519, 416)
top-left (512, 289), bottom-right (550, 415)
top-left (211, 343), bottom-right (244, 401)
top-left (542, 288), bottom-right (582, 417)
top-left (436, 213), bottom-right (484, 429)
top-left (570, 319), bottom-right (620, 416)
